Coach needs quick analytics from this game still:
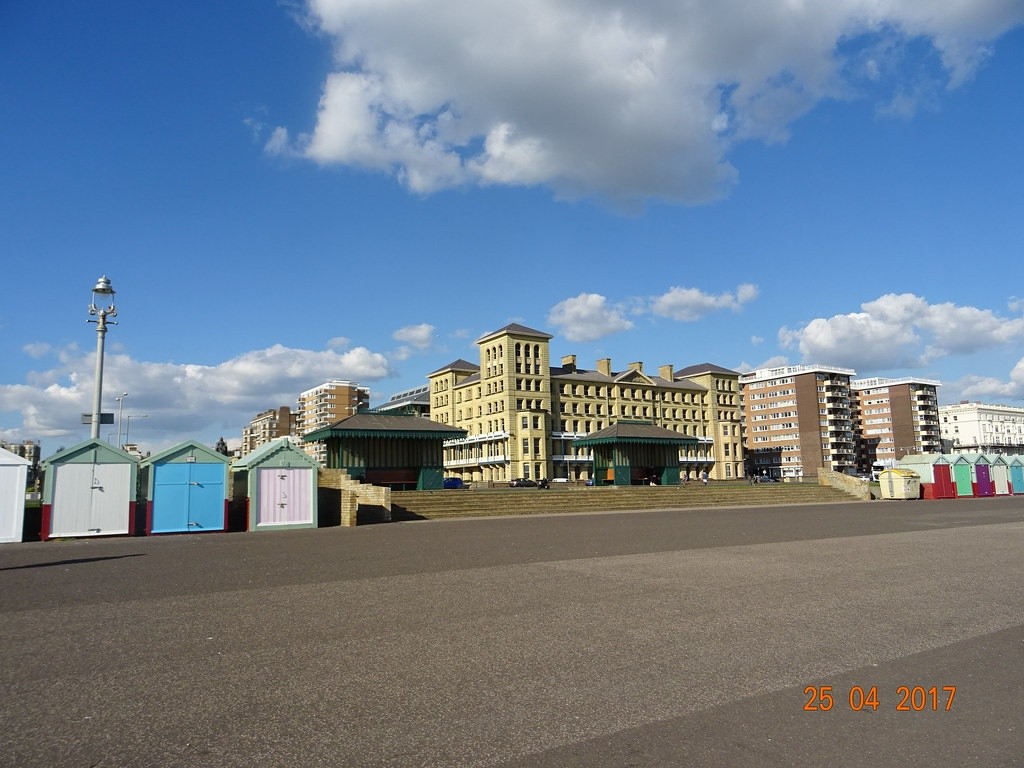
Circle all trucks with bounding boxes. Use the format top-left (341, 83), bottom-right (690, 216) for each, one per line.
top-left (872, 460), bottom-right (892, 482)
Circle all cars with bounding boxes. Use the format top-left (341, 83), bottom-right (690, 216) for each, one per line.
top-left (443, 477), bottom-right (463, 489)
top-left (508, 477), bottom-right (538, 487)
top-left (585, 479), bottom-right (593, 486)
top-left (760, 475), bottom-right (781, 483)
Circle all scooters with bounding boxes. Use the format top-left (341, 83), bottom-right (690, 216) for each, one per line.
top-left (537, 481), bottom-right (549, 489)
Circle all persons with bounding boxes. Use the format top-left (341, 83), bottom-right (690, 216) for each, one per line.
top-left (684, 475), bottom-right (687, 483)
top-left (640, 466), bottom-right (658, 487)
top-left (702, 471), bottom-right (708, 485)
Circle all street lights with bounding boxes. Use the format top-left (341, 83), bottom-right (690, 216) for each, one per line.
top-left (116, 392), bottom-right (128, 449)
top-left (86, 276), bottom-right (119, 439)
top-left (126, 415), bottom-right (148, 446)
